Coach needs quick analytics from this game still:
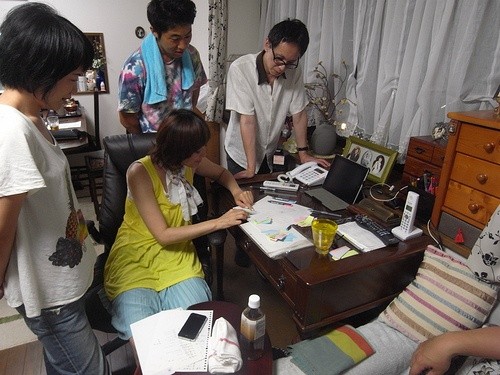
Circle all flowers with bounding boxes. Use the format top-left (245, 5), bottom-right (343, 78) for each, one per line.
top-left (91, 40), bottom-right (105, 70)
top-left (305, 59), bottom-right (357, 127)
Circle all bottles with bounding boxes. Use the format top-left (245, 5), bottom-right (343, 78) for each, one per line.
top-left (100, 82), bottom-right (105, 91)
top-left (239, 294), bottom-right (266, 361)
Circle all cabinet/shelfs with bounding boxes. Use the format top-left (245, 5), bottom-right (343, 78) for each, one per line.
top-left (429, 108), bottom-right (500, 258)
top-left (403, 134), bottom-right (447, 193)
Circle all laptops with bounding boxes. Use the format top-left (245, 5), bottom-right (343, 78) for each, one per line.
top-left (305, 154), bottom-right (369, 212)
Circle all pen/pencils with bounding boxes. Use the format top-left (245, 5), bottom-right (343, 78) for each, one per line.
top-left (308, 209), bottom-right (343, 217)
top-left (273, 197), bottom-right (298, 204)
top-left (250, 186), bottom-right (276, 190)
top-left (267, 200), bottom-right (293, 206)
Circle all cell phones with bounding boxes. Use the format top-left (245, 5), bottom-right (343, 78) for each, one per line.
top-left (177, 312), bottom-right (207, 342)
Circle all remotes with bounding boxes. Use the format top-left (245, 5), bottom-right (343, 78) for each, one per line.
top-left (263, 180), bottom-right (300, 192)
top-left (355, 213), bottom-right (399, 246)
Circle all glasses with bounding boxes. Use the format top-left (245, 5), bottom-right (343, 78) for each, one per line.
top-left (270, 42), bottom-right (299, 69)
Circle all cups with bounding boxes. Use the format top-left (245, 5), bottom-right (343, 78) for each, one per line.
top-left (87, 83), bottom-right (96, 91)
top-left (47, 116), bottom-right (59, 130)
top-left (311, 219), bottom-right (337, 255)
top-left (78, 76), bottom-right (87, 91)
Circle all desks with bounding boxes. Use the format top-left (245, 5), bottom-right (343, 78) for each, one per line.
top-left (57, 105), bottom-right (95, 202)
top-left (131, 299), bottom-right (273, 375)
top-left (208, 170), bottom-right (438, 341)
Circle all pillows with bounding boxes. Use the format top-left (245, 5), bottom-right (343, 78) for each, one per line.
top-left (378, 245), bottom-right (498, 345)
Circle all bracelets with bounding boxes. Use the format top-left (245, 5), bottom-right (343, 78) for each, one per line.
top-left (296, 147), bottom-right (309, 151)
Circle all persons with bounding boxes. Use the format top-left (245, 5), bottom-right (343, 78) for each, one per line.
top-left (0, 3), bottom-right (110, 375)
top-left (118, 0), bottom-right (210, 247)
top-left (102, 108), bottom-right (254, 375)
top-left (223, 18), bottom-right (329, 270)
top-left (409, 326), bottom-right (500, 375)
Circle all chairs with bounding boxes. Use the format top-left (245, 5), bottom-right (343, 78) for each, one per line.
top-left (84, 131), bottom-right (228, 375)
top-left (84, 155), bottom-right (104, 222)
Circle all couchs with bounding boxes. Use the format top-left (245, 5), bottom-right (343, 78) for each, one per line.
top-left (276, 201), bottom-right (500, 375)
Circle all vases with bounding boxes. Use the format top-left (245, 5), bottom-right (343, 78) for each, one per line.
top-left (311, 120), bottom-right (337, 156)
top-left (96, 67), bottom-right (106, 90)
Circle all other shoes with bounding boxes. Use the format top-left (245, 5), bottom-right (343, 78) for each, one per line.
top-left (235, 240), bottom-right (250, 266)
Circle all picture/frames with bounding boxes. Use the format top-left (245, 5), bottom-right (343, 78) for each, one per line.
top-left (341, 135), bottom-right (399, 187)
top-left (71, 32), bottom-right (111, 96)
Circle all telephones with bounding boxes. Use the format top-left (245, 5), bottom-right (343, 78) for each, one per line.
top-left (391, 191), bottom-right (424, 242)
top-left (290, 161), bottom-right (328, 186)
